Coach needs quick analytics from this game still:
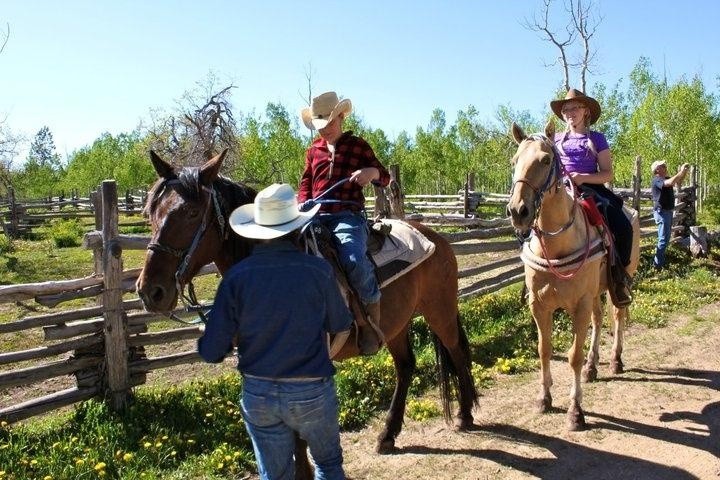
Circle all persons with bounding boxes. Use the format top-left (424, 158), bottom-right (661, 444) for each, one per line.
top-left (194, 180), bottom-right (355, 480)
top-left (649, 160), bottom-right (692, 269)
top-left (293, 90), bottom-right (392, 357)
top-left (546, 86), bottom-right (633, 305)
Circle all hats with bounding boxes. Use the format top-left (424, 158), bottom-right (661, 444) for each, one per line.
top-left (227, 180), bottom-right (321, 242)
top-left (651, 160), bottom-right (666, 172)
top-left (299, 90), bottom-right (352, 132)
top-left (548, 87), bottom-right (602, 126)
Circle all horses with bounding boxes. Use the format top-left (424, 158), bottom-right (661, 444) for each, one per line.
top-left (134, 146), bottom-right (482, 480)
top-left (505, 117), bottom-right (641, 431)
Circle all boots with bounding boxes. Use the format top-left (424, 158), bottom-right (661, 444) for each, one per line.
top-left (617, 265), bottom-right (632, 306)
top-left (359, 297), bottom-right (384, 355)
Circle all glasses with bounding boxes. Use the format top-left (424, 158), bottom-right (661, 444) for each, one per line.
top-left (560, 105), bottom-right (589, 112)
